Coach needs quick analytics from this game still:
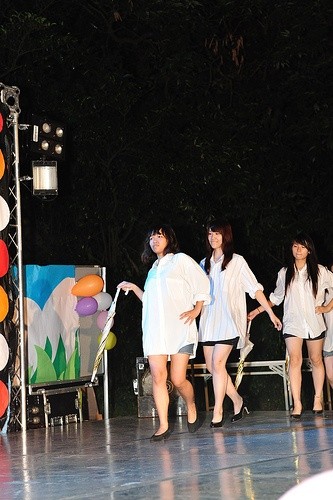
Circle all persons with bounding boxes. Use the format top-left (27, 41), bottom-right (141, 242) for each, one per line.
top-left (117, 223), bottom-right (211, 441)
top-left (247, 233), bottom-right (333, 418)
top-left (199, 219), bottom-right (282, 428)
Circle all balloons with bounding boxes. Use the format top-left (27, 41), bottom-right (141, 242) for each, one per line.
top-left (73, 274), bottom-right (118, 351)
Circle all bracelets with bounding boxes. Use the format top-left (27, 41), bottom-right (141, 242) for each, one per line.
top-left (257, 307), bottom-right (260, 313)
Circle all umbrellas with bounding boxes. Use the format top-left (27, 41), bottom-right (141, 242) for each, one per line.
top-left (90, 281), bottom-right (130, 385)
top-left (234, 321), bottom-right (254, 391)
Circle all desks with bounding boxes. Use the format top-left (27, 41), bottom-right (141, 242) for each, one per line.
top-left (186, 357), bottom-right (333, 412)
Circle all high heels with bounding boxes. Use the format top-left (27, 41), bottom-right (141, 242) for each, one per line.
top-left (231, 398), bottom-right (249, 423)
top-left (290, 406), bottom-right (304, 419)
top-left (150, 427), bottom-right (171, 441)
top-left (313, 409), bottom-right (323, 415)
top-left (210, 409), bottom-right (224, 428)
top-left (187, 403), bottom-right (199, 433)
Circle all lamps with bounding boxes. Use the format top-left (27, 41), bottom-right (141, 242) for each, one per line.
top-left (17, 119), bottom-right (67, 196)
